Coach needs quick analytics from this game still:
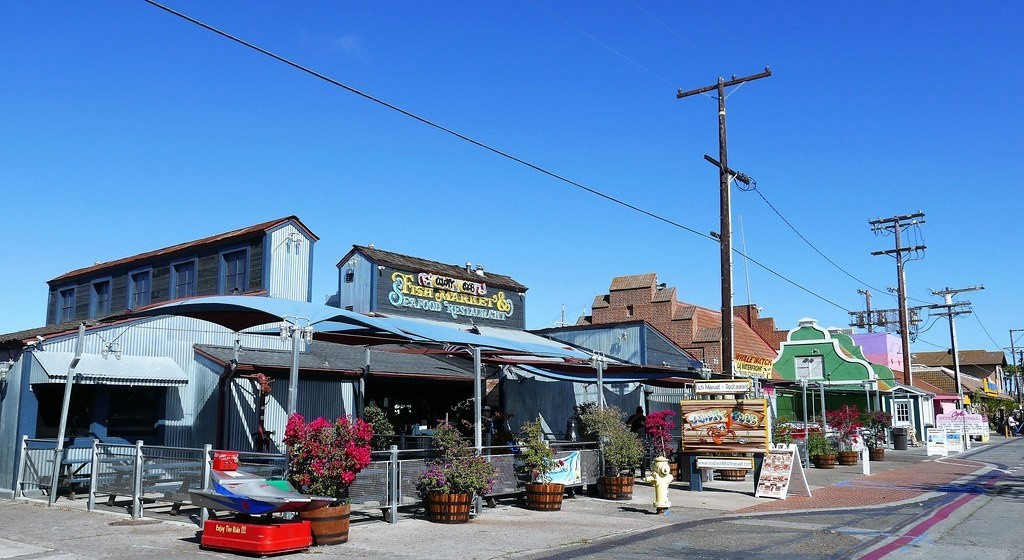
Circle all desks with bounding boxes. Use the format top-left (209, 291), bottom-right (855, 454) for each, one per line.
top-left (168, 466), bottom-right (284, 515)
top-left (107, 461), bottom-right (203, 508)
top-left (47, 458), bottom-right (151, 501)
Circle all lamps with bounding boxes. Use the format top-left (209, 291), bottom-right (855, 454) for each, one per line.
top-left (812, 349), bottom-right (819, 355)
top-left (518, 293), bottom-right (526, 297)
top-left (102, 341), bottom-right (122, 361)
top-left (348, 255), bottom-right (359, 267)
top-left (287, 232), bottom-right (303, 247)
top-left (378, 266), bottom-right (386, 271)
top-left (615, 332), bottom-right (627, 347)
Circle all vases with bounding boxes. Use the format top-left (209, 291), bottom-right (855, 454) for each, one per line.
top-left (526, 482), bottom-right (565, 512)
top-left (669, 463), bottom-right (680, 480)
top-left (299, 505), bottom-right (352, 547)
top-left (839, 451), bottom-right (859, 466)
top-left (423, 493), bottom-right (473, 524)
top-left (869, 449), bottom-right (885, 461)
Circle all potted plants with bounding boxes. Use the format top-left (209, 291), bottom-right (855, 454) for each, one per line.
top-left (579, 403), bottom-right (648, 501)
top-left (714, 453), bottom-right (747, 481)
top-left (807, 431), bottom-right (839, 468)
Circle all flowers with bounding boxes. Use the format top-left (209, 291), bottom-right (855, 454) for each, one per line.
top-left (645, 409), bottom-right (680, 463)
top-left (863, 409), bottom-right (893, 448)
top-left (415, 419), bottom-right (502, 498)
top-left (827, 404), bottom-right (865, 450)
top-left (283, 411), bottom-right (375, 506)
top-left (514, 416), bottom-right (564, 483)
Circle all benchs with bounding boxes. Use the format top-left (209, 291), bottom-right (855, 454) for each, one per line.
top-left (40, 471), bottom-right (187, 515)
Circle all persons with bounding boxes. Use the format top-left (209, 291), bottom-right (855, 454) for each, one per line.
top-left (624, 406), bottom-right (653, 479)
top-left (1007, 413), bottom-right (1021, 436)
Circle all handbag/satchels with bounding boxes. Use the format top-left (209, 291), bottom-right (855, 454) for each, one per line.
top-left (1016, 422), bottom-right (1019, 425)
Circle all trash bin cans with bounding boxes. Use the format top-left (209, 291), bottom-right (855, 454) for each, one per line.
top-left (891, 426), bottom-right (909, 451)
